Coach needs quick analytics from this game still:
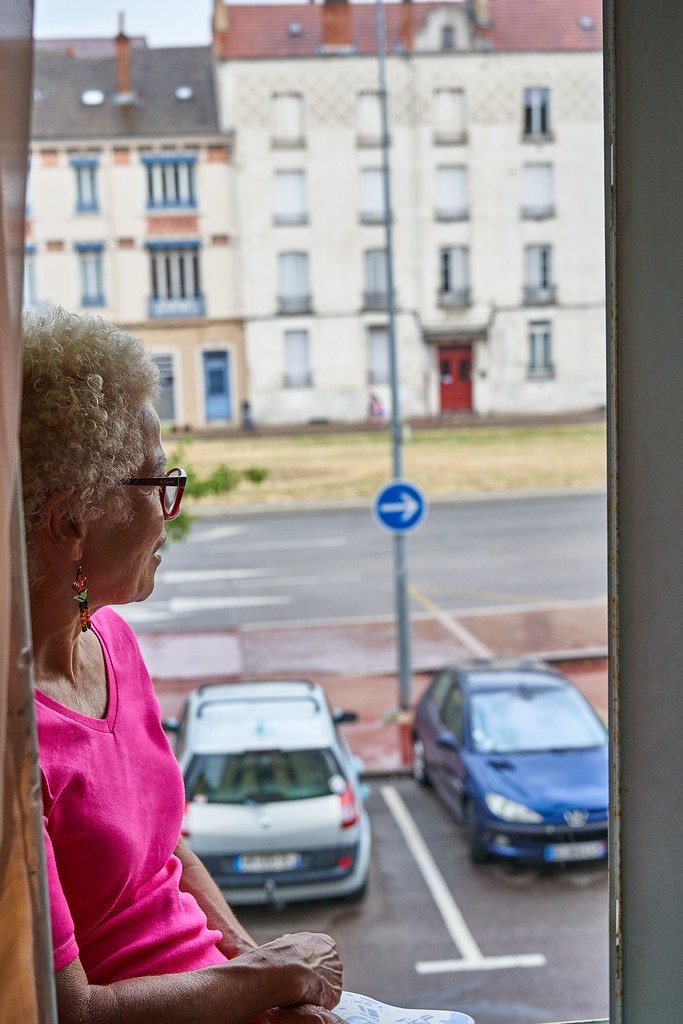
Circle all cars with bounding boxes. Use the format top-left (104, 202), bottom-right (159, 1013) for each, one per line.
top-left (410, 657), bottom-right (609, 871)
top-left (160, 678), bottom-right (373, 913)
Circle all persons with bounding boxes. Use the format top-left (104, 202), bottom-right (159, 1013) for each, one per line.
top-left (22, 306), bottom-right (472, 1024)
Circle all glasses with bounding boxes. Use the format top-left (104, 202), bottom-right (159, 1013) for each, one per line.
top-left (33, 467), bottom-right (188, 521)
